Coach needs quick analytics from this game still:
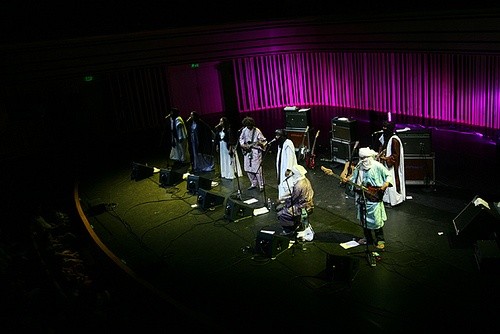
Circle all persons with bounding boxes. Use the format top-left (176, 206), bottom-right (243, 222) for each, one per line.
top-left (277, 165), bottom-right (314, 236)
top-left (378, 121), bottom-right (406, 208)
top-left (214, 117), bottom-right (243, 180)
top-left (274, 129), bottom-right (297, 200)
top-left (343, 147), bottom-right (391, 249)
top-left (239, 117), bottom-right (268, 192)
top-left (186, 111), bottom-right (216, 173)
top-left (169, 108), bottom-right (190, 165)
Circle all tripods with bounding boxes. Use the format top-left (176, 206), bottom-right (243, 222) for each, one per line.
top-left (347, 185), bottom-right (385, 264)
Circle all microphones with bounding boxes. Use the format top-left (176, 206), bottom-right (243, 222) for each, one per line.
top-left (238, 127), bottom-right (244, 131)
top-left (165, 114), bottom-right (170, 118)
top-left (283, 175), bottom-right (292, 182)
top-left (215, 124), bottom-right (221, 128)
top-left (187, 117), bottom-right (191, 122)
top-left (268, 138), bottom-right (276, 144)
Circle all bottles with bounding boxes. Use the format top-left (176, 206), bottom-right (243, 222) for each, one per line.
top-left (268, 198), bottom-right (271, 209)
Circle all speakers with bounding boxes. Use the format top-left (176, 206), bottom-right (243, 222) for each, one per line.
top-left (452, 196), bottom-right (496, 245)
top-left (187, 175), bottom-right (211, 192)
top-left (325, 254), bottom-right (360, 282)
top-left (224, 198), bottom-right (254, 221)
top-left (132, 163), bottom-right (154, 179)
top-left (197, 188), bottom-right (225, 210)
top-left (158, 169), bottom-right (183, 186)
top-left (256, 231), bottom-right (290, 258)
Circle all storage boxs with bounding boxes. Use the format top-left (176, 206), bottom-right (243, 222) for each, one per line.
top-left (330, 138), bottom-right (355, 164)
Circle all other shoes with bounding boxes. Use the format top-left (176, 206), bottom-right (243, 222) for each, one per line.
top-left (358, 238), bottom-right (372, 245)
top-left (280, 230), bottom-right (292, 236)
top-left (376, 244), bottom-right (385, 249)
top-left (260, 187), bottom-right (264, 192)
top-left (384, 203), bottom-right (392, 209)
top-left (248, 186), bottom-right (256, 189)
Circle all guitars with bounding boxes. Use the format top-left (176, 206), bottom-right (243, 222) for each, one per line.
top-left (305, 130), bottom-right (321, 168)
top-left (296, 126), bottom-right (310, 162)
top-left (319, 165), bottom-right (385, 203)
